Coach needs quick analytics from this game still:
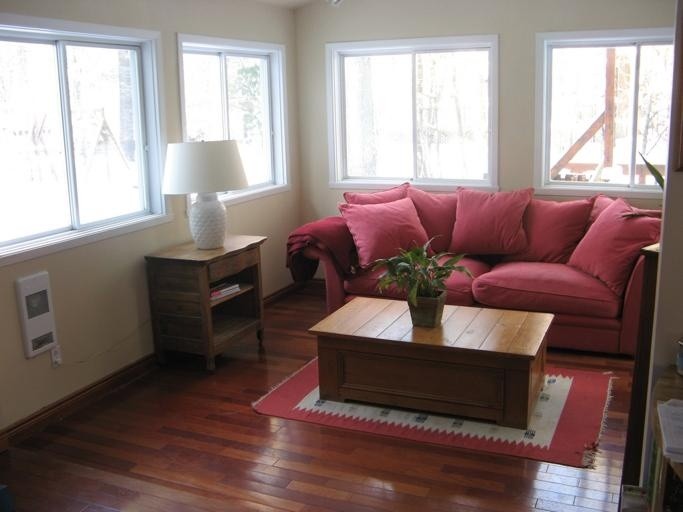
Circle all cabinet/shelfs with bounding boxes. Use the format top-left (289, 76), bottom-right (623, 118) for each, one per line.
top-left (143, 233), bottom-right (269, 371)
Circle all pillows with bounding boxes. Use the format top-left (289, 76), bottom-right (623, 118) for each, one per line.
top-left (342, 256), bottom-right (489, 306)
top-left (342, 180), bottom-right (411, 205)
top-left (566, 195), bottom-right (663, 297)
top-left (447, 186), bottom-right (536, 257)
top-left (401, 184), bottom-right (459, 256)
top-left (507, 196), bottom-right (596, 263)
top-left (337, 197), bottom-right (437, 272)
top-left (472, 260), bottom-right (623, 318)
top-left (586, 190), bottom-right (639, 230)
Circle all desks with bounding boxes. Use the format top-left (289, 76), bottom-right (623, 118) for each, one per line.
top-left (648, 363), bottom-right (683, 512)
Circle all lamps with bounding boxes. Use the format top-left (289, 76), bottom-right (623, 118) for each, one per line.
top-left (160, 138), bottom-right (250, 251)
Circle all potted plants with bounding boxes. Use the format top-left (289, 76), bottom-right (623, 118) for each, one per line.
top-left (361, 234), bottom-right (477, 329)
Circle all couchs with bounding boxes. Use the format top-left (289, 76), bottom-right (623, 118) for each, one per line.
top-left (283, 181), bottom-right (663, 363)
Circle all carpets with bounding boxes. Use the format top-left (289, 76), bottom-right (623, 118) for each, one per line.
top-left (251, 352), bottom-right (621, 470)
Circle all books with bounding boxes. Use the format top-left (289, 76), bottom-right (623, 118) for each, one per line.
top-left (656, 399), bottom-right (683, 463)
top-left (209, 282), bottom-right (241, 301)
